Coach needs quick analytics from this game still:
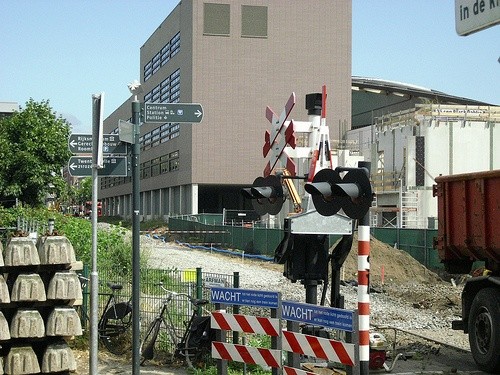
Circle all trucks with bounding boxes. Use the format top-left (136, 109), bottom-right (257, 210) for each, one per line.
top-left (433, 170), bottom-right (500, 374)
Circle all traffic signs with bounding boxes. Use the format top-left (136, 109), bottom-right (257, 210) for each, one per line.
top-left (67, 103), bottom-right (204, 178)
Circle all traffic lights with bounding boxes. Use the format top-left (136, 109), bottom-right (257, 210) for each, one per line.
top-left (242, 175), bottom-right (284, 217)
top-left (304, 167), bottom-right (372, 219)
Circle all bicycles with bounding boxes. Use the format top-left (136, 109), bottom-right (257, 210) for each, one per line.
top-left (139, 278), bottom-right (225, 375)
top-left (65, 274), bottom-right (134, 357)
